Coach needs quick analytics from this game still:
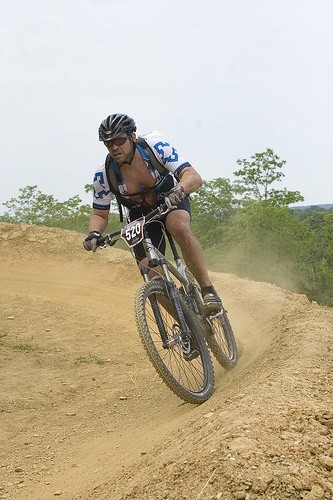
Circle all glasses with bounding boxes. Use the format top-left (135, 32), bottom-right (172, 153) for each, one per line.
top-left (103, 135), bottom-right (129, 148)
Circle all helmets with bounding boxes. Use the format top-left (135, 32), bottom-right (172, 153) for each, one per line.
top-left (98, 114), bottom-right (136, 141)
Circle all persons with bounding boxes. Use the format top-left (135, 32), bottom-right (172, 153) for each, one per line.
top-left (82, 114), bottom-right (222, 362)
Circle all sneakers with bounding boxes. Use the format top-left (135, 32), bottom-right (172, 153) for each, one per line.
top-left (177, 324), bottom-right (200, 361)
top-left (201, 283), bottom-right (222, 321)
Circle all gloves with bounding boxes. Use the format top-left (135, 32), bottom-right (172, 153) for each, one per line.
top-left (164, 185), bottom-right (185, 209)
top-left (83, 231), bottom-right (102, 252)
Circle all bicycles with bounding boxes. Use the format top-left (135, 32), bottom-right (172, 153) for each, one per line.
top-left (96, 186), bottom-right (238, 405)
